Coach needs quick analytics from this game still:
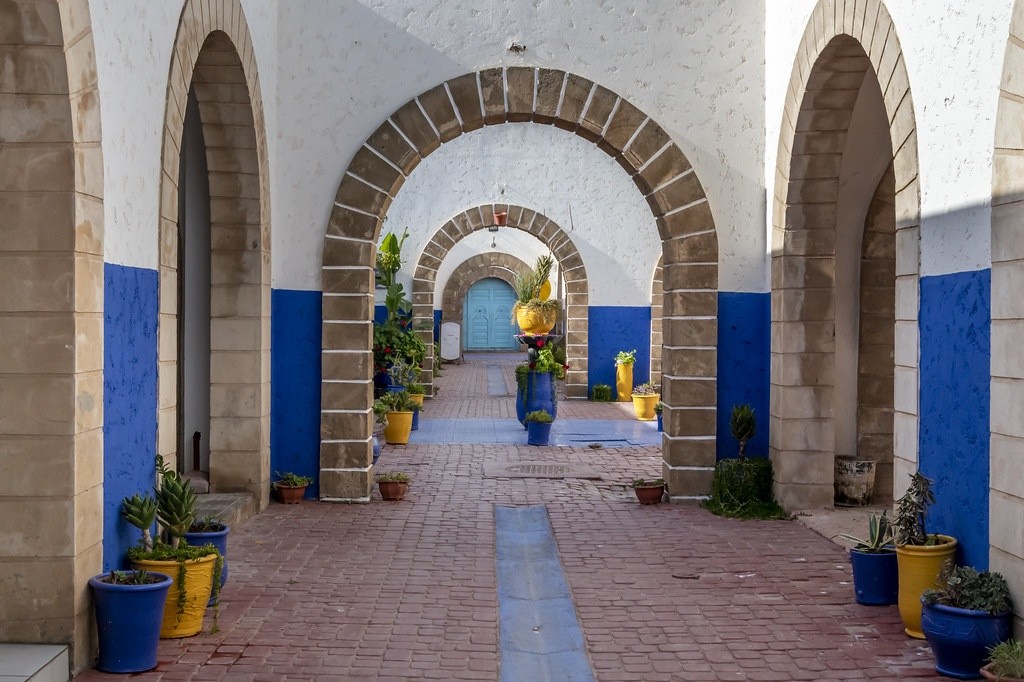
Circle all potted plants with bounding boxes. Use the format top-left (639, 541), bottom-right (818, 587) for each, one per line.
top-left (272, 471), bottom-right (313, 504)
top-left (373, 227), bottom-right (434, 444)
top-left (632, 478), bottom-right (665, 504)
top-left (89, 454), bottom-right (231, 673)
top-left (614, 349), bottom-right (663, 432)
top-left (375, 470), bottom-right (411, 501)
top-left (830, 472), bottom-right (1024, 682)
top-left (511, 254), bottom-right (566, 445)
